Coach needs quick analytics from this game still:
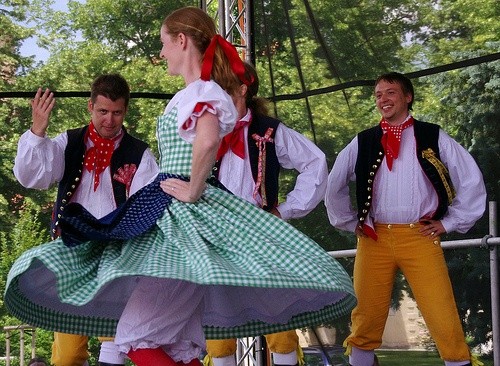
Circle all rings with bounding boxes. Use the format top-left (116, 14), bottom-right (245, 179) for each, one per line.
top-left (172, 187), bottom-right (176, 191)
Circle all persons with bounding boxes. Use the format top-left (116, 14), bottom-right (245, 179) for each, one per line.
top-left (203, 60), bottom-right (328, 366)
top-left (114, 7), bottom-right (256, 366)
top-left (28, 357), bottom-right (49, 366)
top-left (325, 72), bottom-right (487, 366)
top-left (12, 73), bottom-right (160, 366)
